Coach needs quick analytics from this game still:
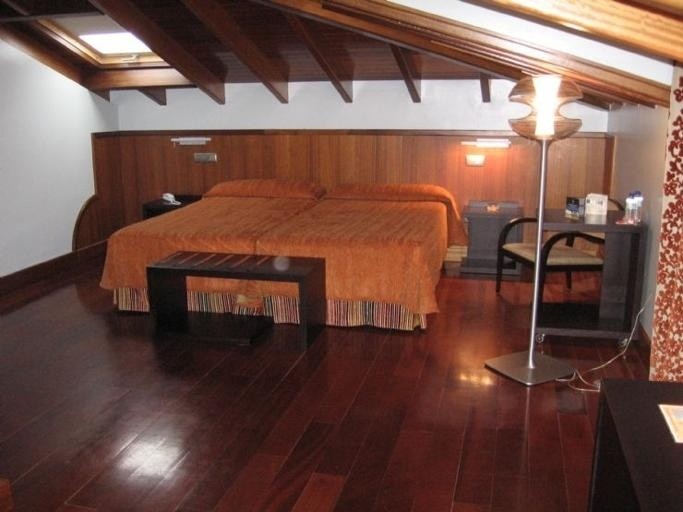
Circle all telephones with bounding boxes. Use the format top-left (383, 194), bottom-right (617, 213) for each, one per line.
top-left (163, 194), bottom-right (175, 202)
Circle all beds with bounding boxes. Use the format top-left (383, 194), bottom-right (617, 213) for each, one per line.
top-left (99, 178), bottom-right (326, 317)
top-left (256, 184), bottom-right (464, 336)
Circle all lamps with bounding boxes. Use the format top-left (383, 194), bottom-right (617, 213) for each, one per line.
top-left (170, 137), bottom-right (211, 146)
top-left (460, 138), bottom-right (511, 150)
top-left (483, 74), bottom-right (584, 386)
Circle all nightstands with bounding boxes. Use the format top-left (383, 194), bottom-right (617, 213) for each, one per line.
top-left (142, 196), bottom-right (201, 219)
top-left (461, 200), bottom-right (524, 276)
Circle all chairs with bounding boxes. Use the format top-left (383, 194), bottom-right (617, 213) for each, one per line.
top-left (496, 199), bottom-right (625, 322)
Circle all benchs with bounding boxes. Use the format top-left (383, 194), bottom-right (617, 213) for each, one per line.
top-left (145, 250), bottom-right (326, 350)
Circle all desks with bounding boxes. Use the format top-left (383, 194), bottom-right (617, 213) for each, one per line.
top-left (536, 208), bottom-right (649, 348)
top-left (587, 376), bottom-right (683, 510)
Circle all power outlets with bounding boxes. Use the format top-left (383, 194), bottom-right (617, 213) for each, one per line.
top-left (467, 154), bottom-right (485, 166)
top-left (194, 152), bottom-right (217, 163)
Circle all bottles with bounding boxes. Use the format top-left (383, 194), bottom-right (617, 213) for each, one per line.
top-left (624, 190), bottom-right (644, 225)
